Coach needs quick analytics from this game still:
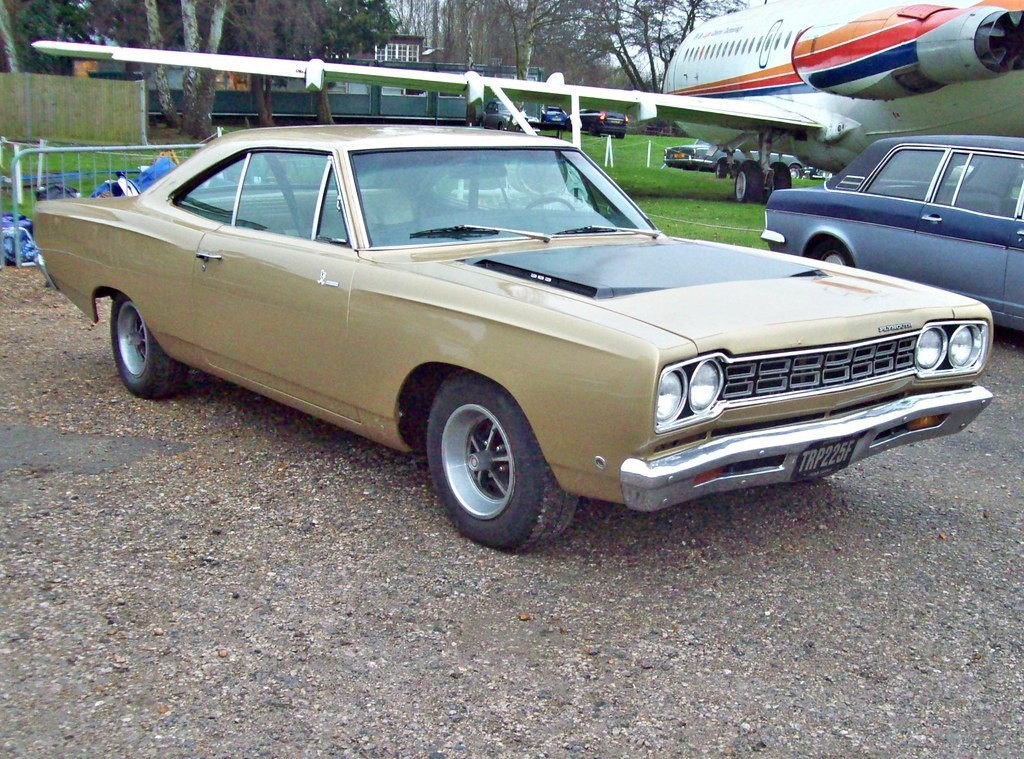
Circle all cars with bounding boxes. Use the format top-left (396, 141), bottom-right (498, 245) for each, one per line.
top-left (761, 134), bottom-right (1024, 334)
top-left (663, 138), bottom-right (806, 180)
top-left (32, 125), bottom-right (996, 554)
top-left (541, 106), bottom-right (570, 131)
top-left (482, 101), bottom-right (540, 132)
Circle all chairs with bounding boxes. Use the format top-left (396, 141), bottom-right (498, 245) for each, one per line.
top-left (199, 187), bottom-right (412, 236)
top-left (957, 159), bottom-right (1011, 215)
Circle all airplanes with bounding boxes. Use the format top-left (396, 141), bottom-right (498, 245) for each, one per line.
top-left (662, 0), bottom-right (1024, 203)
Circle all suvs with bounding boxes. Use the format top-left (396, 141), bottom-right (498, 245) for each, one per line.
top-left (565, 109), bottom-right (629, 139)
